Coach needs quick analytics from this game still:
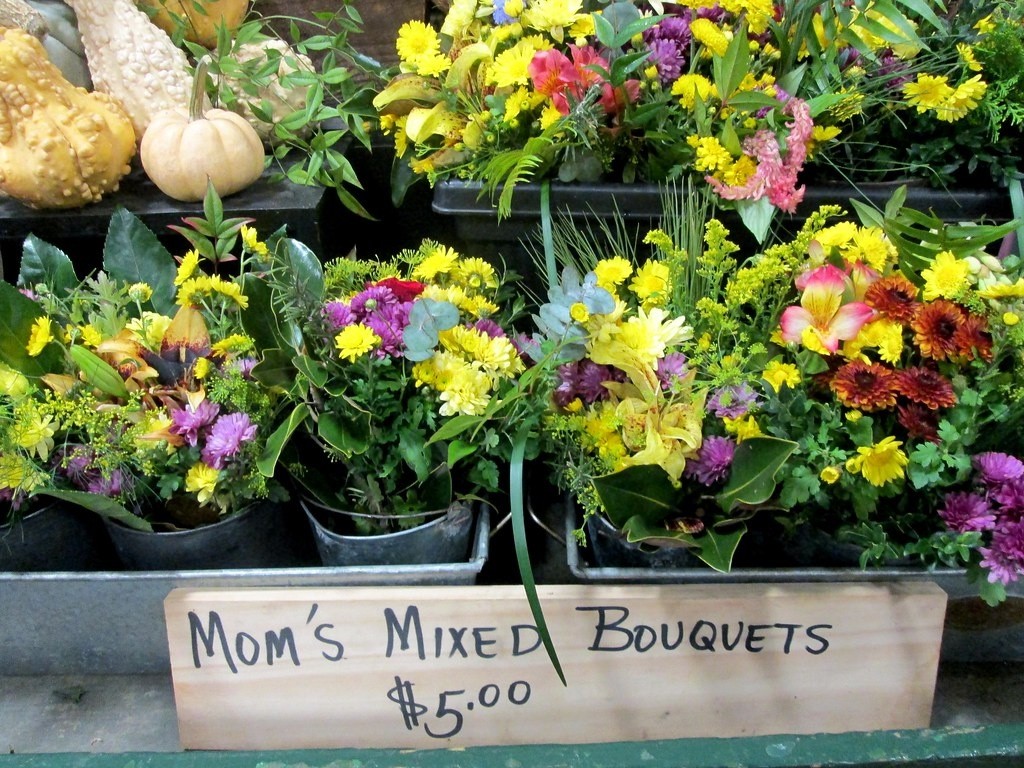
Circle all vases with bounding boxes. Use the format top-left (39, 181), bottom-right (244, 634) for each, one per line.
top-left (584, 497), bottom-right (714, 570)
top-left (786, 505), bottom-right (938, 569)
top-left (0, 464), bottom-right (96, 573)
top-left (298, 489), bottom-right (475, 567)
top-left (94, 467), bottom-right (281, 570)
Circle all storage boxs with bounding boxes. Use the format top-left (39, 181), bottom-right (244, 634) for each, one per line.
top-left (0, 479), bottom-right (491, 680)
top-left (428, 170), bottom-right (1016, 285)
top-left (562, 485), bottom-right (1024, 664)
top-left (0, 124), bottom-right (358, 284)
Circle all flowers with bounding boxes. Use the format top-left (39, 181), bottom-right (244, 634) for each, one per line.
top-left (0, 0), bottom-right (1024, 584)
